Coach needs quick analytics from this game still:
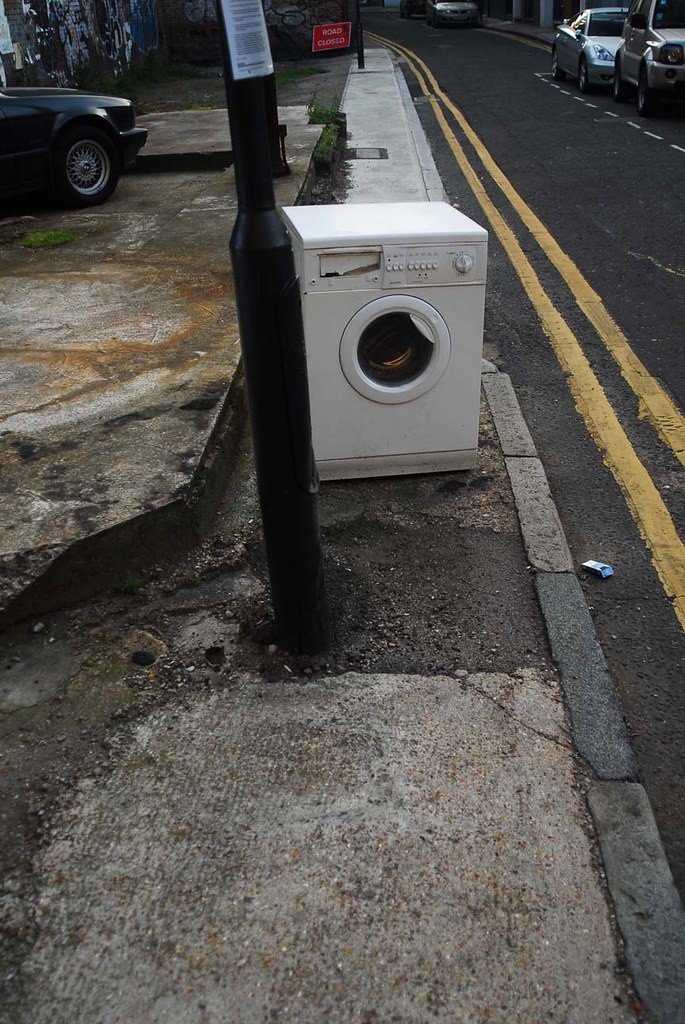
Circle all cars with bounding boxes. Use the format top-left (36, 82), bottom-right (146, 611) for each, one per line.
top-left (400, 0), bottom-right (425, 18)
top-left (425, 0), bottom-right (480, 28)
top-left (0, 87), bottom-right (148, 207)
top-left (551, 6), bottom-right (629, 92)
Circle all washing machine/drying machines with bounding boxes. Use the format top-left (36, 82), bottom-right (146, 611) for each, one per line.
top-left (283, 199), bottom-right (491, 487)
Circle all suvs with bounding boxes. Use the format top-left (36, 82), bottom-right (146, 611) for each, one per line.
top-left (613, 0), bottom-right (685, 116)
